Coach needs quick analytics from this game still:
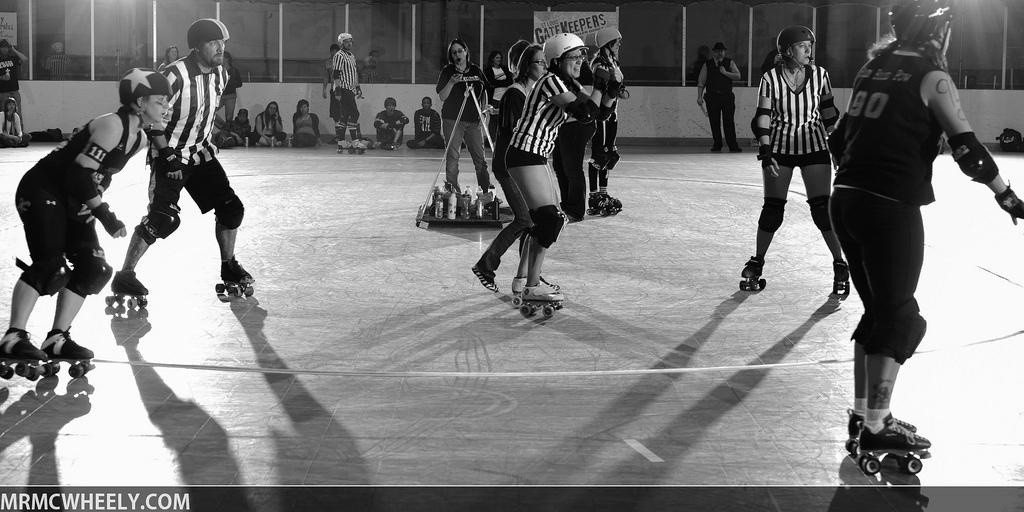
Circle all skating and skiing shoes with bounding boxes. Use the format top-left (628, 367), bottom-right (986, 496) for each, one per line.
top-left (833, 259), bottom-right (850, 294)
top-left (338, 140), bottom-right (353, 153)
top-left (41, 326), bottom-right (94, 378)
top-left (586, 193), bottom-right (614, 216)
top-left (351, 139), bottom-right (368, 154)
top-left (740, 256), bottom-right (766, 290)
top-left (519, 280), bottom-right (564, 317)
top-left (0, 328), bottom-right (49, 381)
top-left (857, 412), bottom-right (931, 475)
top-left (601, 194), bottom-right (622, 214)
top-left (106, 271), bottom-right (148, 309)
top-left (216, 256), bottom-right (255, 296)
top-left (845, 409), bottom-right (916, 452)
top-left (512, 277), bottom-right (527, 308)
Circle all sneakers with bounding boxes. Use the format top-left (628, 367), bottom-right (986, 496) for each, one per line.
top-left (472, 265), bottom-right (498, 292)
top-left (540, 276), bottom-right (560, 290)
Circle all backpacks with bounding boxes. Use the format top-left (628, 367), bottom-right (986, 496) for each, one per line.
top-left (996, 128), bottom-right (1022, 152)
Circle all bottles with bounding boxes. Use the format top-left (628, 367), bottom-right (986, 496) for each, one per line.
top-left (435, 195), bottom-right (444, 219)
top-left (269, 136), bottom-right (276, 146)
top-left (476, 185), bottom-right (483, 198)
top-left (448, 191), bottom-right (457, 219)
top-left (487, 185), bottom-right (496, 201)
top-left (464, 186), bottom-right (473, 203)
top-left (461, 191), bottom-right (471, 219)
top-left (244, 137), bottom-right (250, 146)
top-left (475, 195), bottom-right (484, 219)
top-left (492, 196), bottom-right (499, 219)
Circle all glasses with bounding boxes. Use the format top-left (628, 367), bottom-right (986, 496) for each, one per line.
top-left (563, 55), bottom-right (588, 62)
top-left (451, 48), bottom-right (466, 54)
top-left (530, 61), bottom-right (545, 65)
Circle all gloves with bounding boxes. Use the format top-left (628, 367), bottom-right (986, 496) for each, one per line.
top-left (160, 146), bottom-right (183, 173)
top-left (757, 144), bottom-right (774, 169)
top-left (995, 186), bottom-right (1024, 220)
top-left (91, 202), bottom-right (125, 235)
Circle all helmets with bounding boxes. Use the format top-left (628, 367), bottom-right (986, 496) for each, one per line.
top-left (119, 67), bottom-right (174, 104)
top-left (777, 25), bottom-right (814, 55)
top-left (188, 18), bottom-right (230, 49)
top-left (889, 0), bottom-right (953, 40)
top-left (595, 29), bottom-right (622, 48)
top-left (543, 32), bottom-right (590, 70)
top-left (338, 32), bottom-right (352, 46)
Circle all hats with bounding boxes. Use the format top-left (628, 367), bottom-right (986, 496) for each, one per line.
top-left (712, 42), bottom-right (727, 50)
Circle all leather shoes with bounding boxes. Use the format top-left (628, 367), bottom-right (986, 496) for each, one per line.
top-left (711, 146), bottom-right (722, 151)
top-left (730, 145), bottom-right (742, 152)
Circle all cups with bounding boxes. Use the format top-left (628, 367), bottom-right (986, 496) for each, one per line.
top-left (287, 138), bottom-right (292, 144)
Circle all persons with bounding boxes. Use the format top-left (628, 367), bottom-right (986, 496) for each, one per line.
top-left (433, 26), bottom-right (630, 304)
top-left (213, 32), bottom-right (409, 150)
top-left (406, 96), bottom-right (445, 149)
top-left (0, 97), bottom-right (32, 148)
top-left (738, 23), bottom-right (852, 284)
top-left (676, 44), bottom-right (711, 81)
top-left (157, 45), bottom-right (179, 72)
top-left (44, 41), bottom-right (71, 80)
top-left (0, 38), bottom-right (29, 134)
top-left (696, 41), bottom-right (742, 152)
top-left (109, 16), bottom-right (257, 296)
top-left (0, 65), bottom-right (173, 360)
top-left (825, 3), bottom-right (1024, 453)
